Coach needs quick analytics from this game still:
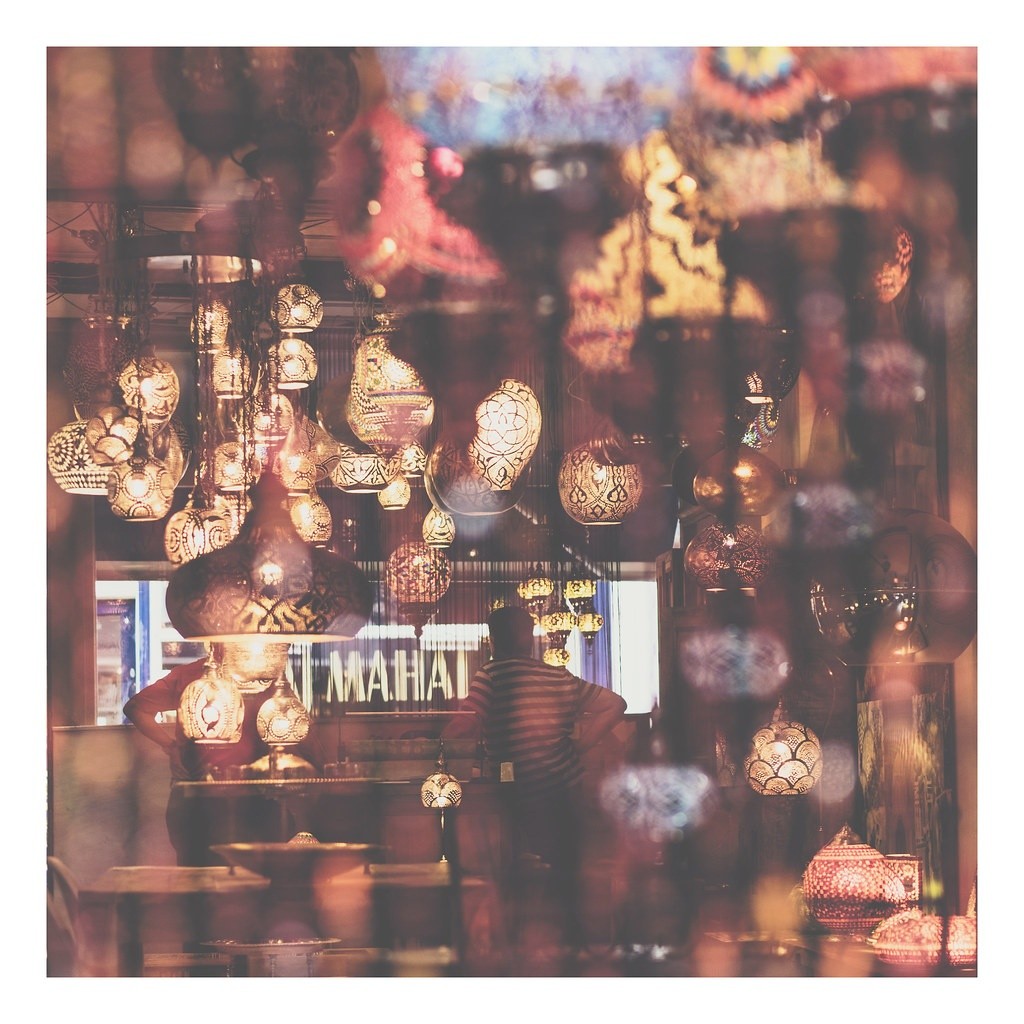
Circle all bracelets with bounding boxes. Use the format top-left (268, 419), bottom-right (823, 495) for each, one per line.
top-left (163, 740), bottom-right (177, 754)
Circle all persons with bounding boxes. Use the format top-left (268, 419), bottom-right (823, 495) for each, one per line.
top-left (122, 652), bottom-right (330, 977)
top-left (437, 606), bottom-right (627, 959)
